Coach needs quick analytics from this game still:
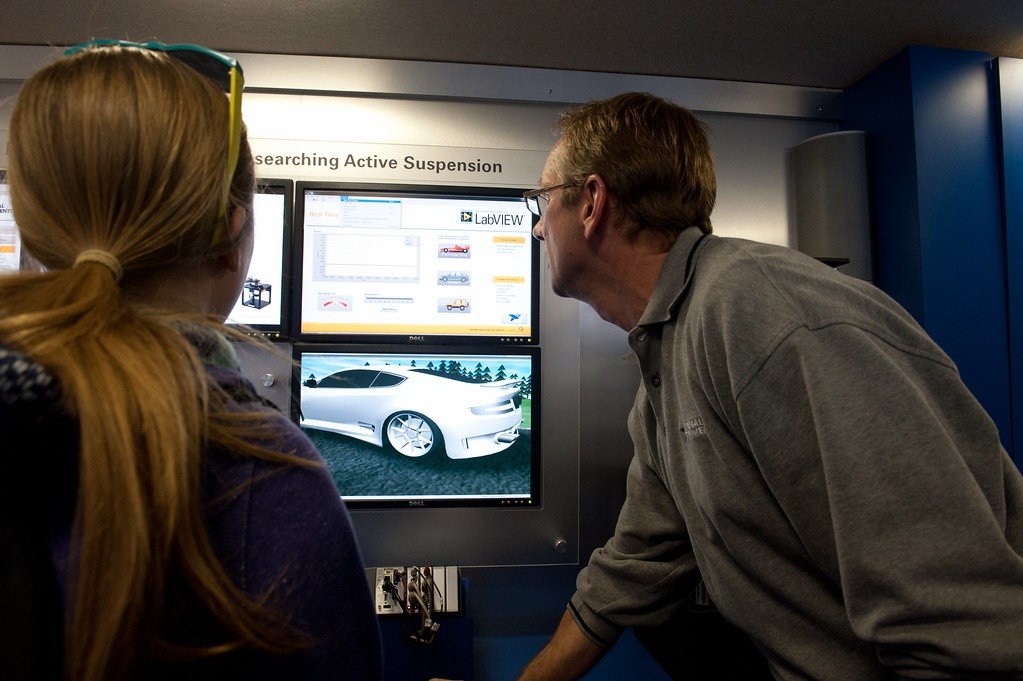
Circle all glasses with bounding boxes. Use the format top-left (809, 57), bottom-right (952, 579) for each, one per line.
top-left (524, 183), bottom-right (574, 216)
top-left (63, 40), bottom-right (244, 247)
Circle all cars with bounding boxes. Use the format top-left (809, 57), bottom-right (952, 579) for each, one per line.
top-left (445, 298), bottom-right (469, 311)
top-left (440, 244), bottom-right (468, 254)
top-left (439, 272), bottom-right (469, 283)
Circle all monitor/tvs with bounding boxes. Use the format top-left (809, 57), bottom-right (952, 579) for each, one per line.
top-left (218, 177), bottom-right (292, 342)
top-left (293, 181), bottom-right (541, 347)
top-left (291, 347), bottom-right (541, 511)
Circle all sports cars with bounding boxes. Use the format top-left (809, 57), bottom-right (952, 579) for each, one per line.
top-left (297, 363), bottom-right (525, 464)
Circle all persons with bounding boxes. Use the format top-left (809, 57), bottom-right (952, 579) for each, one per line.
top-left (0, 39), bottom-right (384, 681)
top-left (519, 90), bottom-right (1023, 681)
